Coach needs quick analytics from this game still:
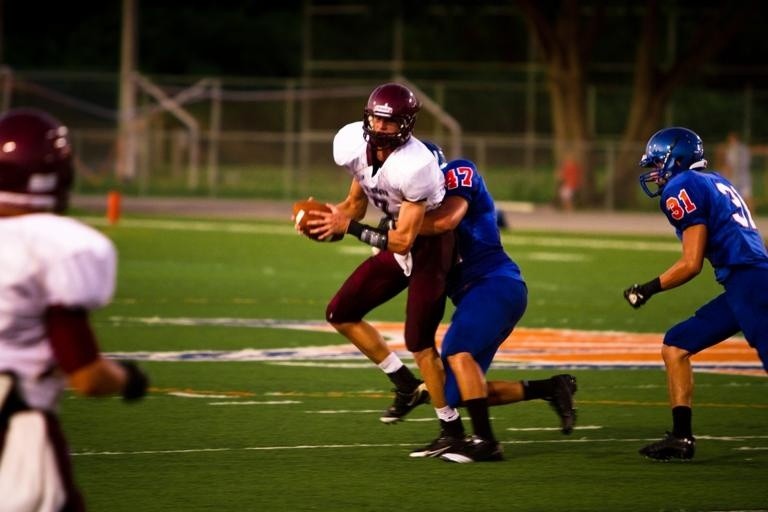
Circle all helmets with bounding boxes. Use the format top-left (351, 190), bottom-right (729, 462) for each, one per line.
top-left (360, 84), bottom-right (418, 150)
top-left (639, 126), bottom-right (709, 198)
top-left (0, 109), bottom-right (77, 212)
top-left (421, 142), bottom-right (449, 171)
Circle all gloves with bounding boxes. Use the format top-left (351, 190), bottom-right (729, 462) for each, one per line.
top-left (119, 360), bottom-right (150, 401)
top-left (623, 284), bottom-right (649, 310)
top-left (377, 217), bottom-right (396, 230)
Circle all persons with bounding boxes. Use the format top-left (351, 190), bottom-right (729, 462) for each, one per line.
top-left (723, 134), bottom-right (754, 214)
top-left (623, 128), bottom-right (768, 461)
top-left (559, 147), bottom-right (581, 212)
top-left (1, 108), bottom-right (150, 512)
top-left (292, 83), bottom-right (474, 460)
top-left (379, 140), bottom-right (578, 435)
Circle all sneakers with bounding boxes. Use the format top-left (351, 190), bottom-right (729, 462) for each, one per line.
top-left (408, 434), bottom-right (465, 458)
top-left (638, 431), bottom-right (696, 465)
top-left (548, 373), bottom-right (578, 435)
top-left (439, 430), bottom-right (504, 464)
top-left (378, 379), bottom-right (432, 425)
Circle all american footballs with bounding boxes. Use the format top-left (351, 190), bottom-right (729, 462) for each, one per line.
top-left (294, 201), bottom-right (344, 242)
